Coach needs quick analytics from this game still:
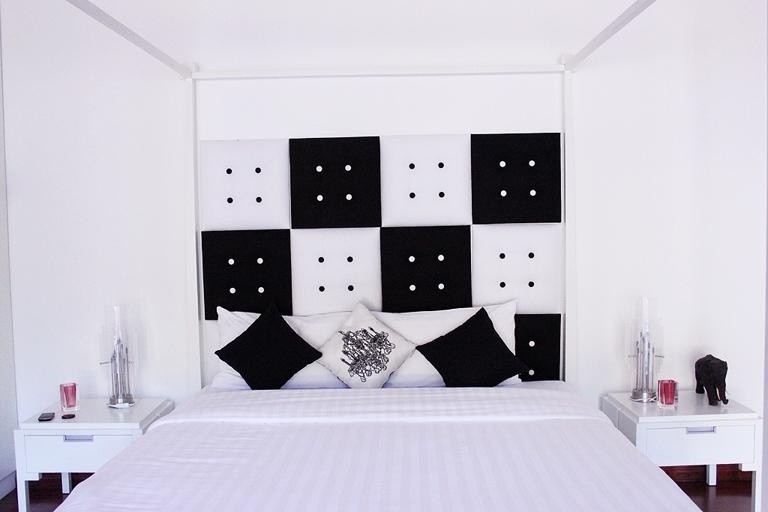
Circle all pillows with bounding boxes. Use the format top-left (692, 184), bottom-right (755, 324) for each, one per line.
top-left (315, 300), bottom-right (419, 389)
top-left (416, 306), bottom-right (530, 387)
top-left (215, 297), bottom-right (322, 390)
top-left (210, 298), bottom-right (523, 390)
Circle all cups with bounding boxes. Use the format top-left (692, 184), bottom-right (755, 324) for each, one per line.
top-left (656, 379), bottom-right (677, 410)
top-left (60, 382), bottom-right (79, 413)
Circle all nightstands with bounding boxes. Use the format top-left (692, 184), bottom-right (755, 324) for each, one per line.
top-left (601, 390), bottom-right (764, 512)
top-left (13, 397), bottom-right (174, 512)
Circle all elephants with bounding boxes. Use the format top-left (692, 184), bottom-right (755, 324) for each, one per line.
top-left (695, 354), bottom-right (728, 406)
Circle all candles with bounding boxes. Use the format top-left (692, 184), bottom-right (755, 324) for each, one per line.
top-left (60, 383), bottom-right (77, 412)
top-left (658, 380), bottom-right (677, 407)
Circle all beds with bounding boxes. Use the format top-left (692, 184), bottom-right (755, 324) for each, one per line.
top-left (53, 386), bottom-right (703, 512)
